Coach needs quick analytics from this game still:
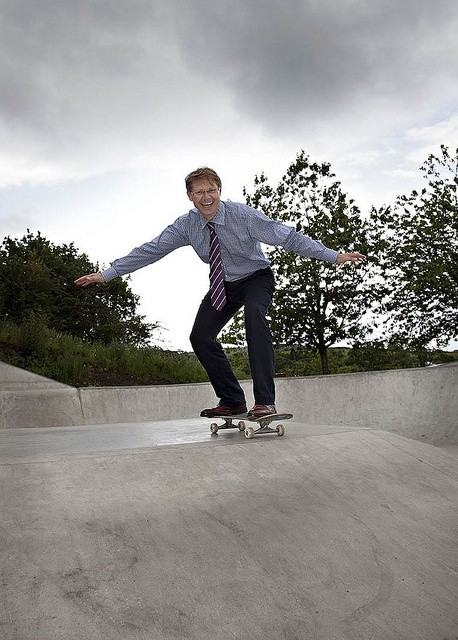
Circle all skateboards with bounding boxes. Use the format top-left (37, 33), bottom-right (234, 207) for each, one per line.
top-left (210, 412), bottom-right (293, 438)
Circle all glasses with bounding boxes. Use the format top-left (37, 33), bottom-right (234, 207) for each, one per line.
top-left (190, 186), bottom-right (219, 196)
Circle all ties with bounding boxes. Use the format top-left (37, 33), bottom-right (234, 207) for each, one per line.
top-left (207, 222), bottom-right (226, 311)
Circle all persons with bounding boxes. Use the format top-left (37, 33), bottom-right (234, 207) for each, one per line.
top-left (73, 167), bottom-right (366, 417)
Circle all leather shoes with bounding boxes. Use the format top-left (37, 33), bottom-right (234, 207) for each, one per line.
top-left (201, 402), bottom-right (247, 417)
top-left (247, 405), bottom-right (276, 420)
